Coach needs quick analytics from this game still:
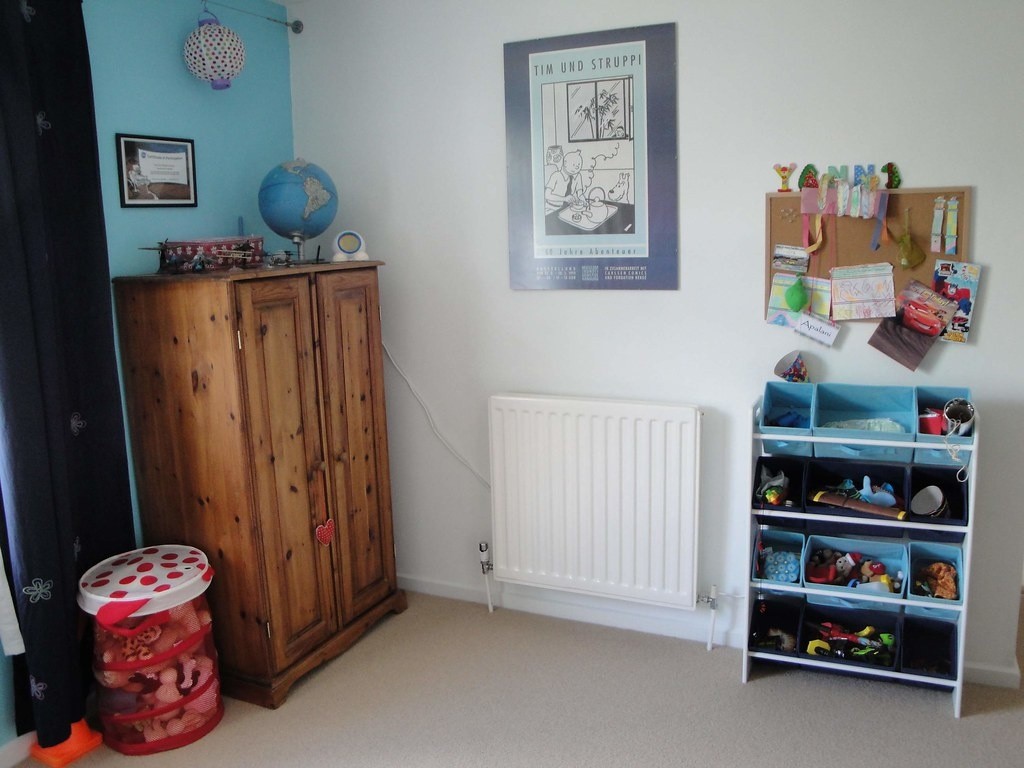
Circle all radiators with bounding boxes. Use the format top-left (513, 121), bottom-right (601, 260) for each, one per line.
top-left (487, 392), bottom-right (704, 612)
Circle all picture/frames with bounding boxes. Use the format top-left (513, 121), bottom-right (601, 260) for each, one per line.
top-left (115, 132), bottom-right (198, 208)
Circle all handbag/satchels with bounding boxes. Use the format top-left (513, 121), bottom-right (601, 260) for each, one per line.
top-left (159, 234), bottom-right (264, 270)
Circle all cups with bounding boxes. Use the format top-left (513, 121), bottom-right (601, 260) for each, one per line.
top-left (942, 399), bottom-right (975, 435)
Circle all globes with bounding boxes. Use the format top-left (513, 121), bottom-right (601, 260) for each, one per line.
top-left (258, 159), bottom-right (339, 267)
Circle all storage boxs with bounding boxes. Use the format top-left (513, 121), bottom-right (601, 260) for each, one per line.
top-left (752, 456), bottom-right (969, 543)
top-left (751, 528), bottom-right (963, 623)
top-left (748, 599), bottom-right (959, 692)
top-left (760, 382), bottom-right (974, 467)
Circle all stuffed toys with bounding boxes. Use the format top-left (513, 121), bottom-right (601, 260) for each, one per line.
top-left (811, 548), bottom-right (903, 593)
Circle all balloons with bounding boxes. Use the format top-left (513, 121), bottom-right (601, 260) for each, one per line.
top-left (785, 279), bottom-right (808, 313)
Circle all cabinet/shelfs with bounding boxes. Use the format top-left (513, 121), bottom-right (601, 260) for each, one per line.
top-left (112, 260), bottom-right (407, 710)
top-left (741, 381), bottom-right (980, 718)
